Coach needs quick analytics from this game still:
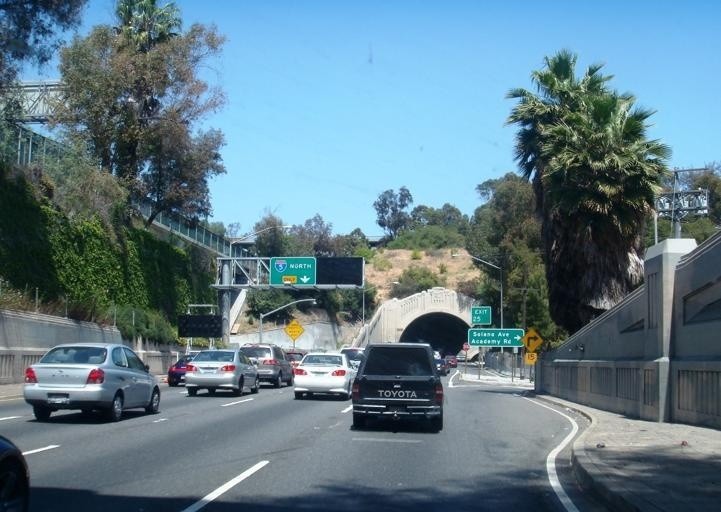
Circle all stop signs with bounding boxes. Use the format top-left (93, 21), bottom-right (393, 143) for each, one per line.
top-left (461, 342), bottom-right (470, 351)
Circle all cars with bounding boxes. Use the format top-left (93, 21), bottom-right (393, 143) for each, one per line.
top-left (284, 348), bottom-right (308, 375)
top-left (431, 348), bottom-right (464, 377)
top-left (237, 342), bottom-right (293, 389)
top-left (20, 341), bottom-right (161, 421)
top-left (0, 434), bottom-right (29, 512)
top-left (182, 349), bottom-right (260, 397)
top-left (339, 343), bottom-right (366, 376)
top-left (167, 354), bottom-right (197, 387)
top-left (292, 352), bottom-right (355, 399)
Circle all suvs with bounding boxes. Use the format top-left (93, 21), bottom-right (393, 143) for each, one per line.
top-left (349, 342), bottom-right (444, 433)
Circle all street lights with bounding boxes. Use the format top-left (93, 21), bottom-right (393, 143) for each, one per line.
top-left (228, 224), bottom-right (294, 257)
top-left (257, 297), bottom-right (317, 343)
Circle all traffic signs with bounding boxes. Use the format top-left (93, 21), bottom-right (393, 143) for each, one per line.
top-left (471, 304), bottom-right (492, 326)
top-left (268, 256), bottom-right (316, 287)
top-left (452, 253), bottom-right (503, 355)
top-left (465, 327), bottom-right (525, 349)
top-left (524, 352), bottom-right (537, 365)
